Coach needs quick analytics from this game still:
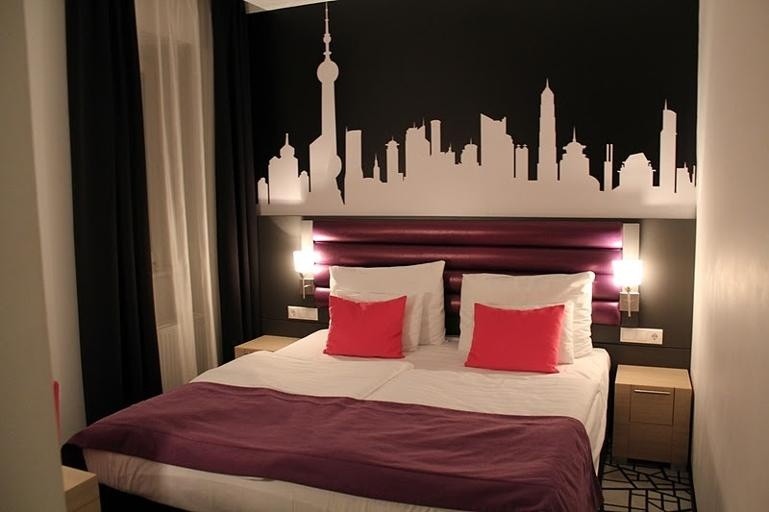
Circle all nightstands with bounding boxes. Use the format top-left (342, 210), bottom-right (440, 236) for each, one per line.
top-left (233, 334), bottom-right (300, 360)
top-left (611, 364), bottom-right (693, 473)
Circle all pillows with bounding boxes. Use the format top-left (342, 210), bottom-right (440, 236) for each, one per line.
top-left (459, 271), bottom-right (595, 374)
top-left (323, 261), bottom-right (446, 359)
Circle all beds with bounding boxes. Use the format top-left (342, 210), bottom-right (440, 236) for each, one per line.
top-left (61, 221), bottom-right (641, 511)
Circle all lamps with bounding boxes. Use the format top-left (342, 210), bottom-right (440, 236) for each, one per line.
top-left (292, 251), bottom-right (317, 297)
top-left (611, 260), bottom-right (644, 317)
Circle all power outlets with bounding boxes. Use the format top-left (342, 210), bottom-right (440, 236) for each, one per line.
top-left (650, 328), bottom-right (663, 345)
